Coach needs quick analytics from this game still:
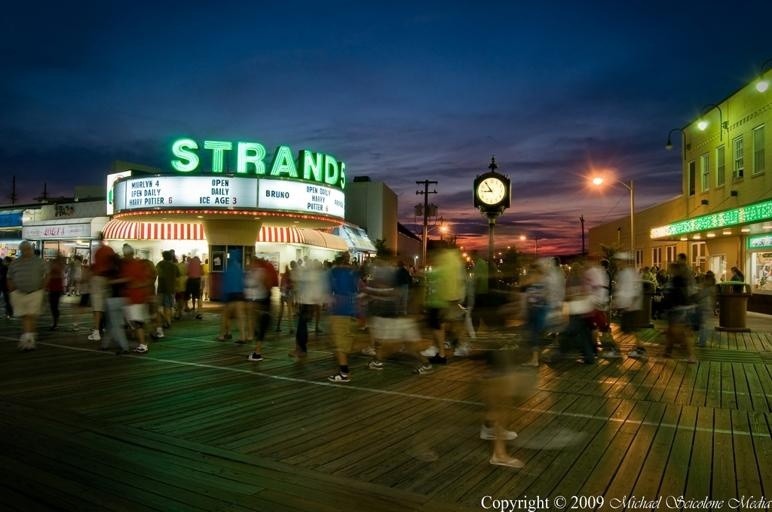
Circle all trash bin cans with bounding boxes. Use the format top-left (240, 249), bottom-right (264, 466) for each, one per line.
top-left (621, 280), bottom-right (654, 330)
top-left (715, 281), bottom-right (753, 333)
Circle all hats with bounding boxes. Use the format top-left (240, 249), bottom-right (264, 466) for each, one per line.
top-left (611, 253), bottom-right (631, 260)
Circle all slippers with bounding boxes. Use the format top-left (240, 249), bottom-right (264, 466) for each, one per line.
top-left (490, 455), bottom-right (524, 469)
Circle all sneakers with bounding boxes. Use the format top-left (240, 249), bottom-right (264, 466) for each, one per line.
top-left (17, 309), bottom-right (202, 354)
top-left (481, 422), bottom-right (518, 440)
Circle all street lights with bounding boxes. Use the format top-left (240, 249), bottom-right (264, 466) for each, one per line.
top-left (590, 173), bottom-right (637, 267)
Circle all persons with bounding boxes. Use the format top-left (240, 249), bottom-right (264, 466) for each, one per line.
top-left (1, 230), bottom-right (745, 384)
